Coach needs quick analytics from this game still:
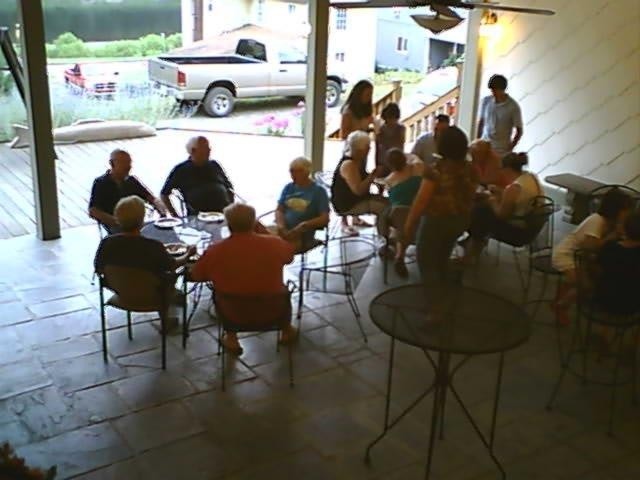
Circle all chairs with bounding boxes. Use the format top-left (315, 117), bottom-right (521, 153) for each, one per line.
top-left (547, 248), bottom-right (639, 437)
top-left (172, 188), bottom-right (245, 217)
top-left (90, 207), bottom-right (153, 285)
top-left (257, 207), bottom-right (327, 291)
top-left (297, 233), bottom-right (386, 342)
top-left (207, 280), bottom-right (296, 392)
top-left (98, 264), bottom-right (187, 369)
top-left (496, 204), bottom-right (561, 291)
top-left (585, 183), bottom-right (639, 213)
top-left (519, 196), bottom-right (576, 330)
top-left (313, 166), bottom-right (518, 267)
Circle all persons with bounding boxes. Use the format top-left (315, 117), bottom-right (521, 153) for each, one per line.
top-left (331, 74), bottom-right (544, 277)
top-left (160, 136), bottom-right (235, 217)
top-left (94, 196), bottom-right (196, 334)
top-left (191, 204), bottom-right (298, 352)
top-left (269, 157), bottom-right (329, 254)
top-left (88, 150), bottom-right (166, 236)
top-left (592, 209), bottom-right (640, 324)
top-left (550, 189), bottom-right (635, 326)
top-left (395, 125), bottom-right (477, 327)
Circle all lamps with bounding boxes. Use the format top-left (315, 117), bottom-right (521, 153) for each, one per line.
top-left (410, 11), bottom-right (462, 35)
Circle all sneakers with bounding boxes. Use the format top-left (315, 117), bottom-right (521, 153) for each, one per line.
top-left (219, 337), bottom-right (243, 356)
top-left (395, 260), bottom-right (407, 278)
top-left (279, 325), bottom-right (298, 346)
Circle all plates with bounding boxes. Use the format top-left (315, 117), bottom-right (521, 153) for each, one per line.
top-left (163, 242), bottom-right (188, 257)
top-left (154, 217), bottom-right (183, 228)
top-left (197, 211), bottom-right (225, 223)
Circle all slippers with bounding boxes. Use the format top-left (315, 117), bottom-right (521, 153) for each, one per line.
top-left (340, 225), bottom-right (359, 236)
top-left (352, 219), bottom-right (373, 227)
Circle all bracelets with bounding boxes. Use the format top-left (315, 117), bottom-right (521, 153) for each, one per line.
top-left (297, 221), bottom-right (306, 233)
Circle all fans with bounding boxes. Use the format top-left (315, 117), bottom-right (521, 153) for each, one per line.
top-left (330, 0), bottom-right (555, 17)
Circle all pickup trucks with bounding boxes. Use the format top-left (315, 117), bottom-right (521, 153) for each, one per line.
top-left (62, 63), bottom-right (124, 101)
top-left (146, 38), bottom-right (349, 118)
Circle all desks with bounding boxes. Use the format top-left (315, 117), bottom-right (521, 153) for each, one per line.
top-left (365, 283), bottom-right (532, 480)
top-left (140, 216), bottom-right (233, 332)
